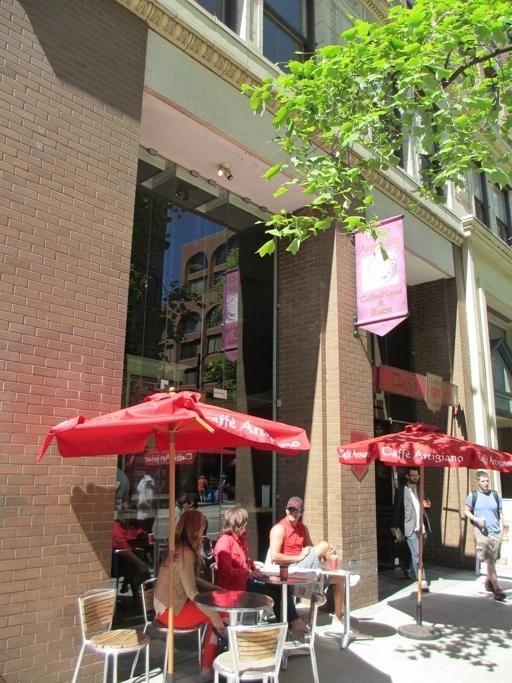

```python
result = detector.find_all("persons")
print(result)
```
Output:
[462,471,507,602]
[212,506,319,635]
[266,494,356,638]
[154,510,232,683]
[111,468,210,622]
[391,467,432,592]
[222,474,231,501]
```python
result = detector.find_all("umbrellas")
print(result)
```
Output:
[34,385,310,682]
[337,420,512,627]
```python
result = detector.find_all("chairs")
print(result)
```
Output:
[212,622,288,683]
[282,592,327,683]
[68,585,150,683]
[141,577,204,672]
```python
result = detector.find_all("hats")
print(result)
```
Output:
[284,495,304,512]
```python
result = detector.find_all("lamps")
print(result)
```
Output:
[216,164,233,180]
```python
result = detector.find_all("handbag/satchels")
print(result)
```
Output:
[315,571,336,612]
[212,621,230,656]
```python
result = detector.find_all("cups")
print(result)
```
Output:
[330,555,338,571]
[280,565,289,581]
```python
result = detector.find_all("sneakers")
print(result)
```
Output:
[330,619,358,633]
[484,577,494,592]
[494,588,506,600]
[421,580,429,590]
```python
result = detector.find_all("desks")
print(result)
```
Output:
[321,569,359,650]
[249,571,319,640]
[193,590,275,626]
